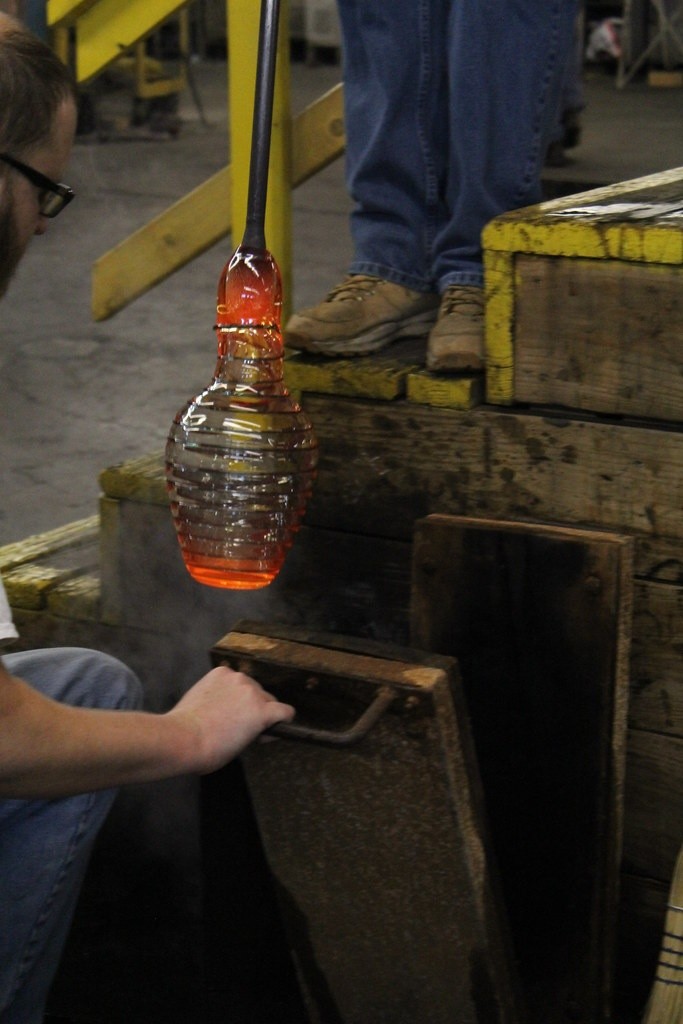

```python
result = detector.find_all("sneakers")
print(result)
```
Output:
[284,273,441,355]
[429,284,486,373]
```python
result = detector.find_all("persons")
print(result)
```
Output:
[0,8,298,1024]
[282,0,624,375]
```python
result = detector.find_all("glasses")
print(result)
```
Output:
[0,150,75,220]
